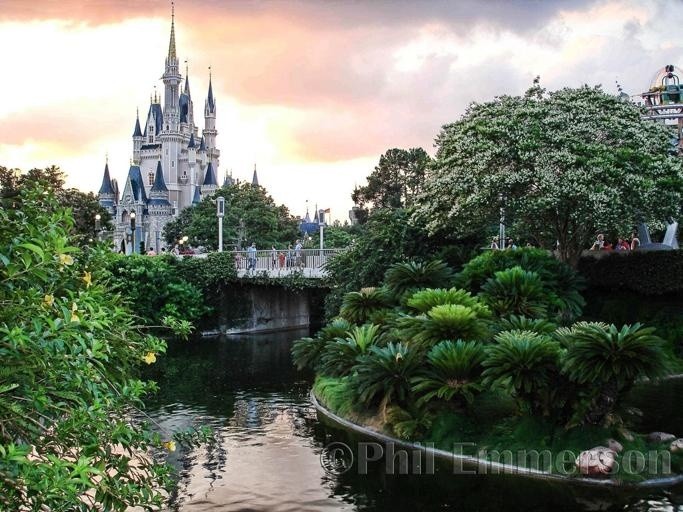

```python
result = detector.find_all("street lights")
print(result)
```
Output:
[130,212,136,253]
[317,208,325,265]
[94,214,101,242]
[215,195,224,253]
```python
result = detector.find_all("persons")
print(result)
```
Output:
[146,245,180,255]
[600,240,613,252]
[589,234,604,250]
[630,226,640,251]
[491,236,499,249]
[615,237,627,251]
[507,239,514,249]
[245,238,305,275]
